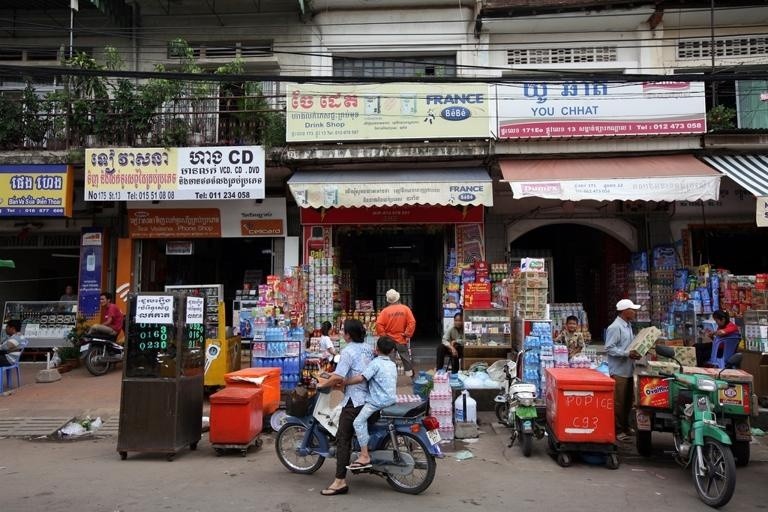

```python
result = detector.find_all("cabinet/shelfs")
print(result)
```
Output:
[503,266,548,353]
[462,307,512,398]
[653,248,675,328]
[628,251,650,336]
[1,301,76,359]
[376,278,413,313]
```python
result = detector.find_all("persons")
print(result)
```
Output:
[553,316,585,360]
[338,337,397,471]
[376,289,416,377]
[60,286,77,301]
[693,309,739,368]
[79,291,123,360]
[307,329,321,348]
[315,317,375,497]
[436,313,463,374]
[0,319,26,367]
[604,298,642,443]
[320,320,337,367]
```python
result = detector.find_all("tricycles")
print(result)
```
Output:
[631,344,759,507]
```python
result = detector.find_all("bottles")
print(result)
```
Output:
[432,372,449,392]
[438,425,454,440]
[488,274,508,282]
[431,409,452,425]
[429,387,452,408]
[491,263,508,274]
[254,327,304,342]
[554,344,570,368]
[303,358,330,384]
[540,335,554,398]
[253,317,267,329]
[532,323,550,336]
[280,375,302,392]
[524,336,540,396]
[251,354,306,374]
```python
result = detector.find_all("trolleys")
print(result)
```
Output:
[543,367,619,470]
[209,387,263,456]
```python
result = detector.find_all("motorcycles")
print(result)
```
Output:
[275,377,444,494]
[79,315,126,376]
[487,348,544,457]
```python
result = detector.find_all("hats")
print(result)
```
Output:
[615,297,643,313]
[384,288,402,304]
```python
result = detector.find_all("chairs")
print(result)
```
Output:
[0,339,29,395]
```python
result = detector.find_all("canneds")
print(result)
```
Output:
[337,312,380,324]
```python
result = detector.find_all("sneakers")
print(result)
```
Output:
[625,424,636,435]
[615,431,634,444]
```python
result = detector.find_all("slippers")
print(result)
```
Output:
[319,485,351,496]
[344,459,375,464]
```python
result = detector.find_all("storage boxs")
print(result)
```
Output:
[208,387,264,445]
[544,366,617,445]
[225,367,282,416]
